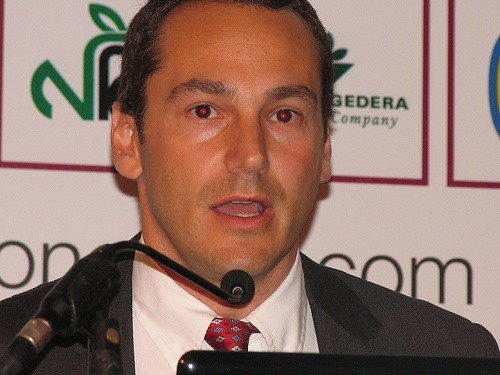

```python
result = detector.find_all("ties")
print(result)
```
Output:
[204,317,260,353]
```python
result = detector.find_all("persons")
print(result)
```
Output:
[1,1,500,375]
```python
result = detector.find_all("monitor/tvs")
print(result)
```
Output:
[176,350,500,374]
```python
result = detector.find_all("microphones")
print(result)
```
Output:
[112,239,255,306]
